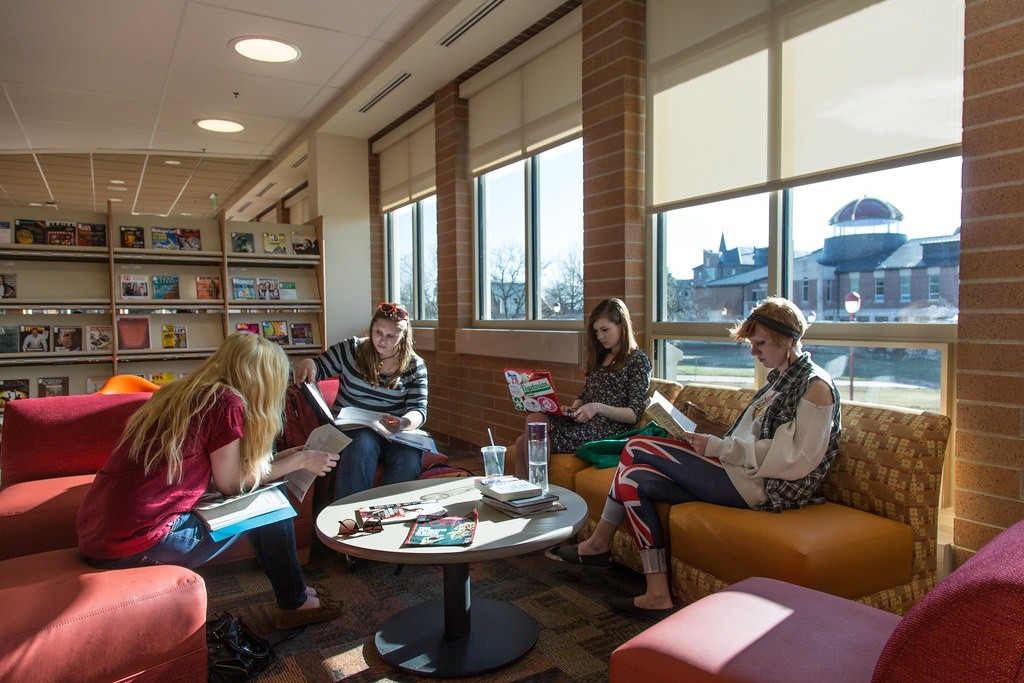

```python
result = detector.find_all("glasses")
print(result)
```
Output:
[379,301,409,318]
[337,516,384,534]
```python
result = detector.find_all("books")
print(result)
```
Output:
[643,390,697,441]
[474,475,567,518]
[303,382,438,454]
[404,509,478,546]
[0,219,320,418]
[359,500,447,523]
[193,480,298,542]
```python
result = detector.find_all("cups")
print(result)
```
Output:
[481,445,507,477]
[526,422,550,492]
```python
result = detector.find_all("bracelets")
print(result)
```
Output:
[573,408,577,410]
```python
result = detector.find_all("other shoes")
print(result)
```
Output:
[313,587,331,597]
[274,597,346,631]
[346,554,360,572]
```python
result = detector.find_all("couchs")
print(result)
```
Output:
[0,377,467,563]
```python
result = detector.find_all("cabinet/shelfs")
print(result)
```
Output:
[0,199,328,427]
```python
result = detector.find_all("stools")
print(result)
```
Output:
[0,550,207,683]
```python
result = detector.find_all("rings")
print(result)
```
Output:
[692,440,693,444]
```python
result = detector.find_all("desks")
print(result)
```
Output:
[316,477,589,676]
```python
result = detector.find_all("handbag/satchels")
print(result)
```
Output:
[575,420,668,469]
[206,610,276,683]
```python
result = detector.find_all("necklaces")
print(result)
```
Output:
[380,345,399,360]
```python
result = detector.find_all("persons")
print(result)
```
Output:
[0,275,14,296]
[22,328,47,352]
[557,298,841,621]
[303,239,311,249]
[262,282,274,292]
[515,298,652,479]
[208,281,218,297]
[76,331,341,629]
[296,302,428,499]
[58,331,81,351]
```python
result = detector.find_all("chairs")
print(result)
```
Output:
[504,378,684,490]
[610,518,1024,683]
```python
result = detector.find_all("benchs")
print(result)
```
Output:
[577,383,951,618]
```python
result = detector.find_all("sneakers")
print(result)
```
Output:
[609,596,673,623]
[544,543,613,569]
